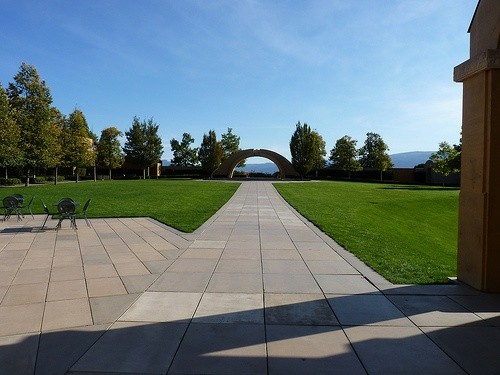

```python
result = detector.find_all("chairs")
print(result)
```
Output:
[0,192,93,233]
